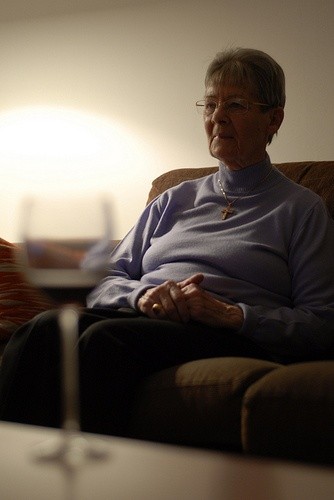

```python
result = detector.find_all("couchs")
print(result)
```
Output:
[147,160,334,458]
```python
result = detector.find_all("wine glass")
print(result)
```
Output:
[17,191,115,474]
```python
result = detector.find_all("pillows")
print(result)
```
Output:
[0,238,56,329]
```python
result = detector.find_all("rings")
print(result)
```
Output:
[152,304,161,313]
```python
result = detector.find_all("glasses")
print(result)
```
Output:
[195,98,270,116]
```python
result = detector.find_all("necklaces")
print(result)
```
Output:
[218,168,273,221]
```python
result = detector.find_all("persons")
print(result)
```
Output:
[0,48,334,439]
